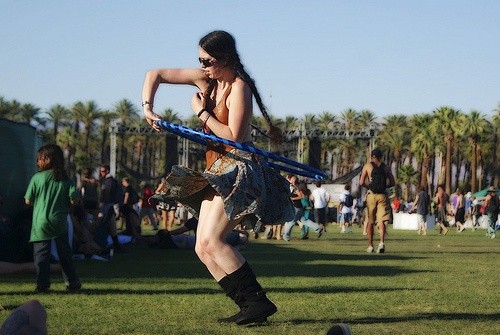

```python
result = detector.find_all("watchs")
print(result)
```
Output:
[142,100,151,106]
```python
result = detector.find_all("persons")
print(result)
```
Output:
[142,31,292,326]
[69,162,500,258]
[24,144,80,293]
[359,149,394,254]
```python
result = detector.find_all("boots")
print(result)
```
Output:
[217,276,249,324]
[226,262,277,324]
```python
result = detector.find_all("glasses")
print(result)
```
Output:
[198,58,218,67]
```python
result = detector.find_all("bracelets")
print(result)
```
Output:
[201,113,210,124]
[198,109,207,118]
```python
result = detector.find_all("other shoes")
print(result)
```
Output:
[67,283,81,294]
[316,227,323,238]
[276,235,281,240]
[377,243,385,253]
[366,247,374,252]
[254,231,258,239]
[459,228,465,232]
[34,286,47,293]
[272,233,275,238]
[443,229,448,235]
[301,232,308,240]
